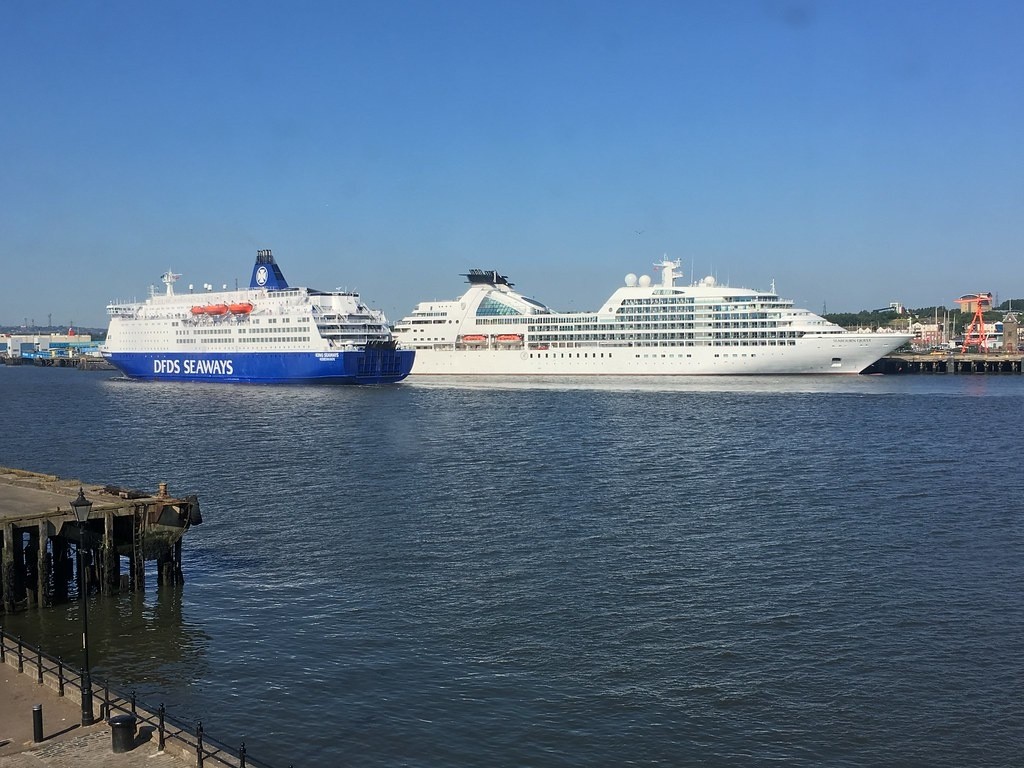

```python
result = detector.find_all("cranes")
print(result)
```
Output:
[952,292,992,354]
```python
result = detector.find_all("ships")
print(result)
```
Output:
[390,254,917,374]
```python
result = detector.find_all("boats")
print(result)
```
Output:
[100,249,416,385]
